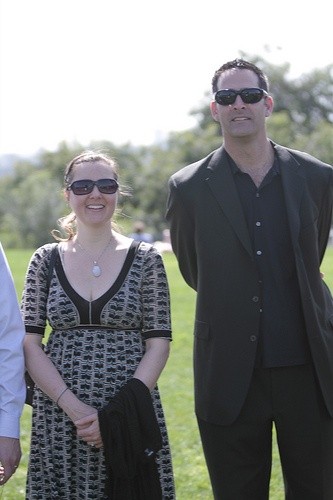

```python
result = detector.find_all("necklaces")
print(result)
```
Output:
[75,236,114,277]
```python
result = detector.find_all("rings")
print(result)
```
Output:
[14,465,18,468]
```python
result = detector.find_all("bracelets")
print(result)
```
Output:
[56,387,69,404]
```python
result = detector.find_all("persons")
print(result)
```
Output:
[0,241,26,485]
[166,58,333,500]
[22,150,175,500]
[128,223,153,244]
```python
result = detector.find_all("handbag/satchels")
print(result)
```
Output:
[24,243,59,406]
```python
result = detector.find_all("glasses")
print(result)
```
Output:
[213,87,269,106]
[66,178,119,195]
